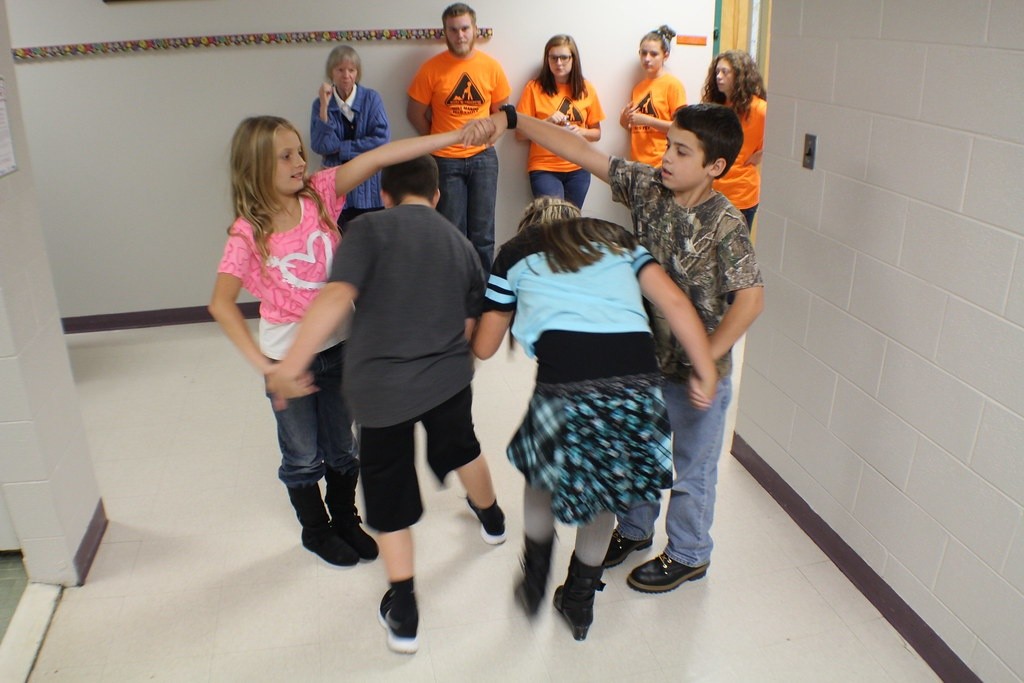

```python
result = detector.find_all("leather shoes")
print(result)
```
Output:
[626,553,710,593]
[602,530,654,570]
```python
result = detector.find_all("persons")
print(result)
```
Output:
[207,115,487,655]
[309,3,768,285]
[470,103,766,641]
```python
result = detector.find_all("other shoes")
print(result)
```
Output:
[465,494,506,545]
[380,588,420,654]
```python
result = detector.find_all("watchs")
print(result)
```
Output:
[498,104,517,129]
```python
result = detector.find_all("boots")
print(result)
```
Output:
[553,550,607,641]
[514,534,555,620]
[324,473,378,563]
[287,481,359,568]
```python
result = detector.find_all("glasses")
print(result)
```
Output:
[548,54,573,63]
